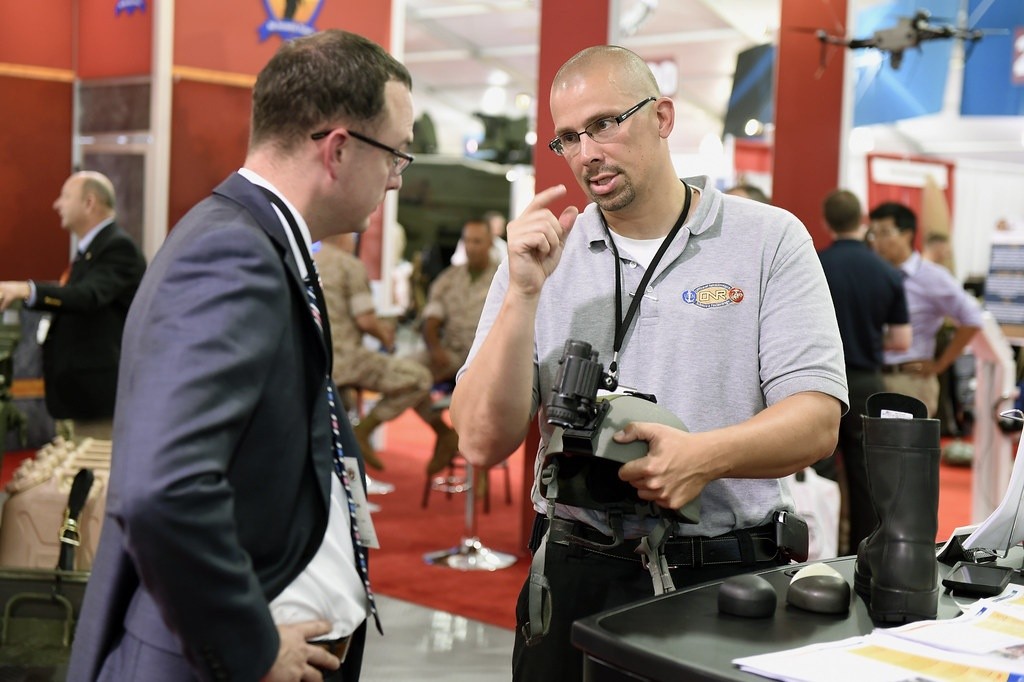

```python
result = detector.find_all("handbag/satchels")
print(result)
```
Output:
[0,592,77,682]
[936,533,1024,567]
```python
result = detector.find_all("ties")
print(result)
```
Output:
[69,250,82,271]
[304,277,384,635]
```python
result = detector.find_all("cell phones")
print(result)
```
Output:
[941,561,1014,595]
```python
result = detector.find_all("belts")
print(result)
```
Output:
[885,362,910,375]
[548,517,779,569]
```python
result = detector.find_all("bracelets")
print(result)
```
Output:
[24,280,31,303]
[937,362,944,374]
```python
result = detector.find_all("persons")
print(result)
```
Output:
[312,211,506,476]
[450,45,851,682]
[68,32,414,682]
[726,185,983,556]
[0,171,147,419]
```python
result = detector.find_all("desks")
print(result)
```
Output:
[567,541,1024,682]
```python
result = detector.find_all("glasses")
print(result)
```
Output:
[311,129,414,176]
[548,97,657,158]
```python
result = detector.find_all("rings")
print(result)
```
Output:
[0,293,2,300]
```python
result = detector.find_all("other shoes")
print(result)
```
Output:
[361,450,383,470]
[427,430,459,475]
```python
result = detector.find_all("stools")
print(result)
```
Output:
[334,384,517,572]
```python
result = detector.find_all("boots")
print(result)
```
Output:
[853,392,940,623]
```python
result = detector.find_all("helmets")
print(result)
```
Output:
[538,396,703,526]
